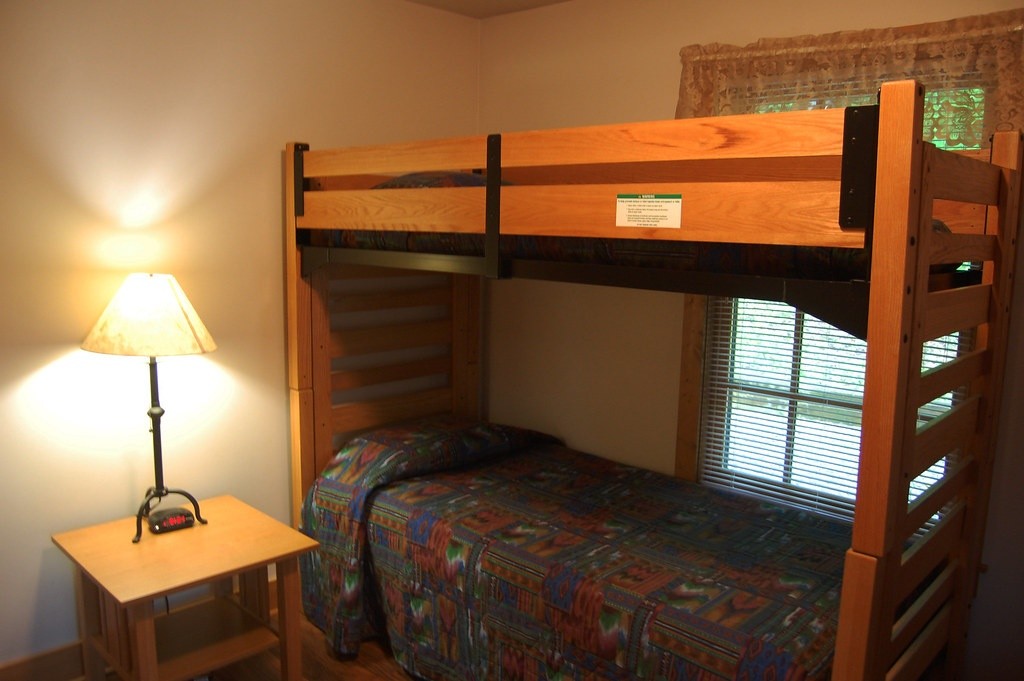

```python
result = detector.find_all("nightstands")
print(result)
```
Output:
[52,494,319,681]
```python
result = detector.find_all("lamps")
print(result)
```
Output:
[82,270,220,546]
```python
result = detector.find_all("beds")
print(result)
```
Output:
[281,78,1024,681]
